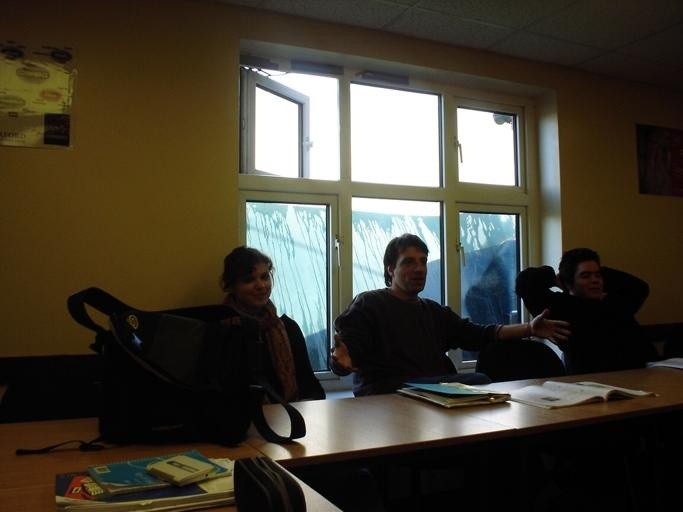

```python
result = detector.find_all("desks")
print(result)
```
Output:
[0,365,682,511]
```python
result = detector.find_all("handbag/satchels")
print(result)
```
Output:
[68,287,268,446]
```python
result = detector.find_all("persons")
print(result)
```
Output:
[221,247,326,400]
[330,234,572,397]
[516,248,661,372]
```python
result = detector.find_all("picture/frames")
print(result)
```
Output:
[635,122,683,198]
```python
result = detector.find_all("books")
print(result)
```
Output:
[511,381,661,410]
[54,449,239,512]
[396,381,512,410]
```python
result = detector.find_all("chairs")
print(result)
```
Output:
[476,335,573,382]
